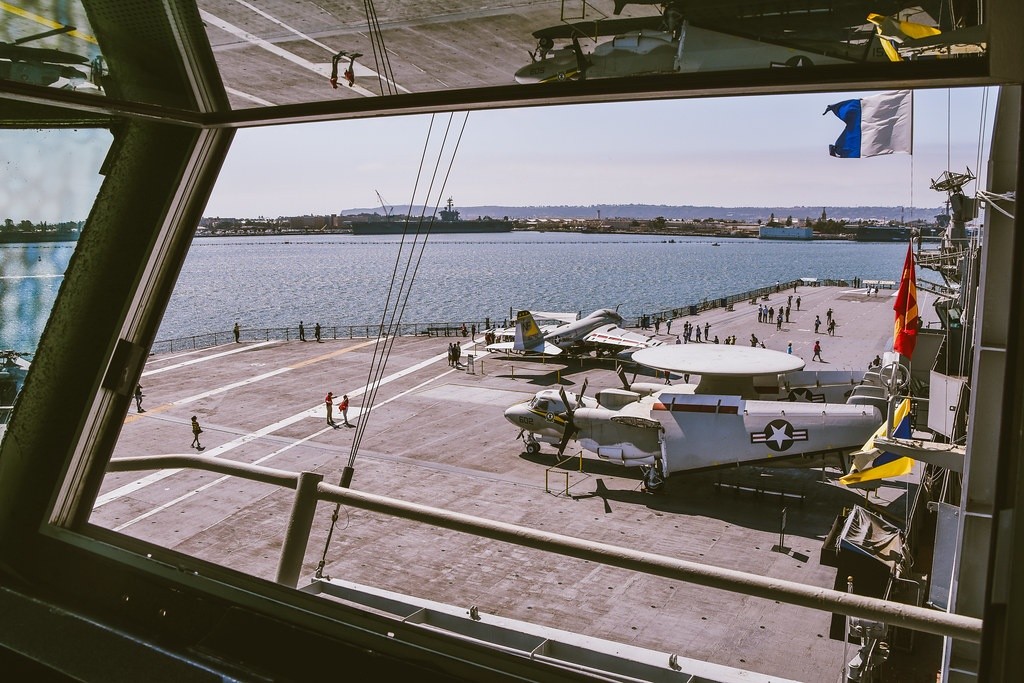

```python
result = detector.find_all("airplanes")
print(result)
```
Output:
[503,382,666,466]
[480,305,669,356]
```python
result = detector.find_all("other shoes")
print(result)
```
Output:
[191,444,195,447]
[327,421,335,424]
[343,421,348,424]
[197,443,201,445]
[137,406,141,412]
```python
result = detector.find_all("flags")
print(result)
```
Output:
[839,394,915,485]
[867,5,942,62]
[892,239,919,362]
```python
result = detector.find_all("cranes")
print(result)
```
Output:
[375,190,395,218]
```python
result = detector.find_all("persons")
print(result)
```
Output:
[461,323,469,337]
[299,321,307,342]
[641,279,880,385]
[325,392,337,425]
[234,323,241,343]
[315,323,321,342]
[135,382,147,413]
[330,51,363,89]
[190,415,203,448]
[341,394,349,425]
[472,325,476,343]
[447,342,462,367]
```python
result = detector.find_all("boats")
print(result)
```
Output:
[438,196,460,221]
[919,201,953,243]
[758,225,817,242]
[857,223,911,241]
